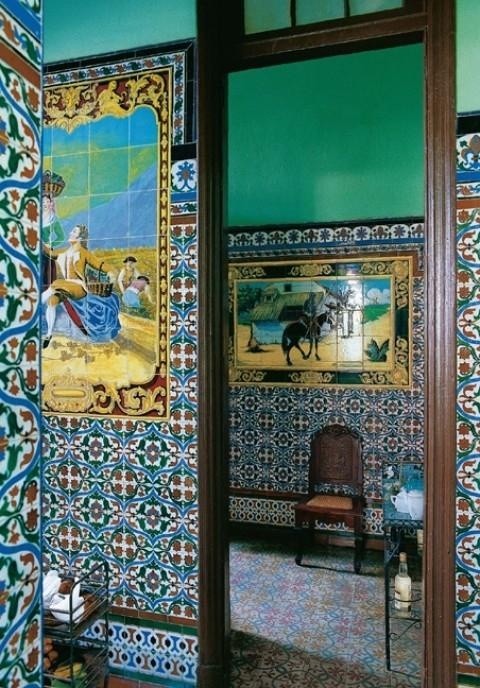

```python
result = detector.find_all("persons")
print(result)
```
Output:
[116,253,141,295]
[42,191,65,290]
[299,289,319,324]
[121,272,158,310]
[38,222,119,352]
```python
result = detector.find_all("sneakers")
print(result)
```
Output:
[42,334,53,350]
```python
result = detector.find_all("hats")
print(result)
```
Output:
[122,256,138,263]
[136,276,151,285]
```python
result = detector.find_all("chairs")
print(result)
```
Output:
[293,424,365,573]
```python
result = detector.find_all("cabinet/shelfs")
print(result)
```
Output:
[43,563,111,688]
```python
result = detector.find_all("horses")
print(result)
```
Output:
[282,310,333,366]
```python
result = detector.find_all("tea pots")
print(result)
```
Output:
[389,486,407,514]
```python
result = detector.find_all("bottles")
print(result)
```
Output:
[404,488,424,520]
[392,550,414,619]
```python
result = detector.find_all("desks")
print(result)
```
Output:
[381,462,426,671]
